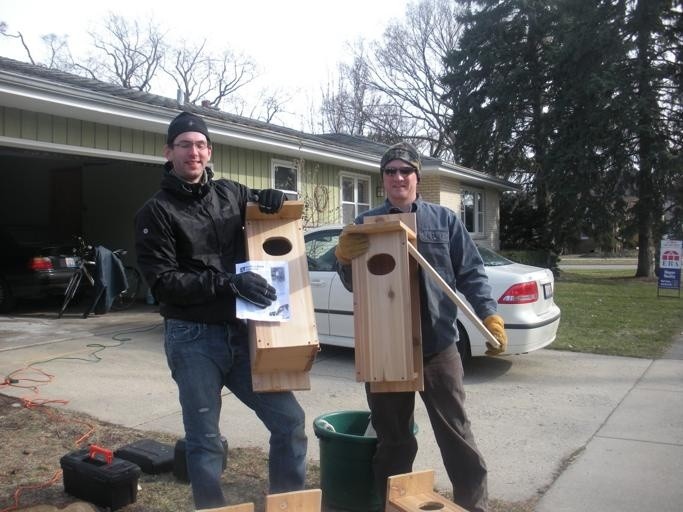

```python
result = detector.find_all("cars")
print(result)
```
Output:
[0,241,82,313]
[304,224,561,366]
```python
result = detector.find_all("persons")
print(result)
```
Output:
[335,141,508,511]
[134,112,307,509]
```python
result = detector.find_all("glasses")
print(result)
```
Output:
[385,166,416,176]
[173,142,209,150]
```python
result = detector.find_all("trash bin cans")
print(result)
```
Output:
[313,410,419,512]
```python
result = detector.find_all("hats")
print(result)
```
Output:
[380,141,421,182]
[168,112,210,144]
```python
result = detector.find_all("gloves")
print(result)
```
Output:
[335,225,370,262]
[254,189,285,215]
[483,315,508,356]
[228,271,277,309]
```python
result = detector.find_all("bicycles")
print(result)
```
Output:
[58,231,142,319]
